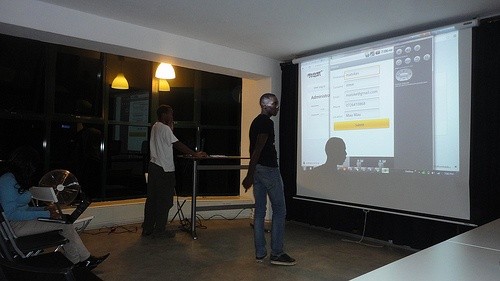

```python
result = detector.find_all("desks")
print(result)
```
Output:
[446,219,500,252]
[347,241,500,281]
[177,153,272,241]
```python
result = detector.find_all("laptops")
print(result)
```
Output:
[40,198,94,224]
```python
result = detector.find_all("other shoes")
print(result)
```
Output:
[84,252,110,272]
[141,231,176,239]
[256,253,268,263]
[270,253,297,265]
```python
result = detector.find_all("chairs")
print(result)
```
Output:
[0,204,71,281]
[30,186,95,239]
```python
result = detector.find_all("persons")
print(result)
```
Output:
[77,123,102,199]
[141,105,205,238]
[242,93,296,266]
[0,160,111,272]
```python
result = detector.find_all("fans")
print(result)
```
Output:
[38,168,81,210]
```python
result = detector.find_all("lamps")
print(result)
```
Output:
[154,62,177,81]
[110,72,129,90]
[158,79,171,92]
